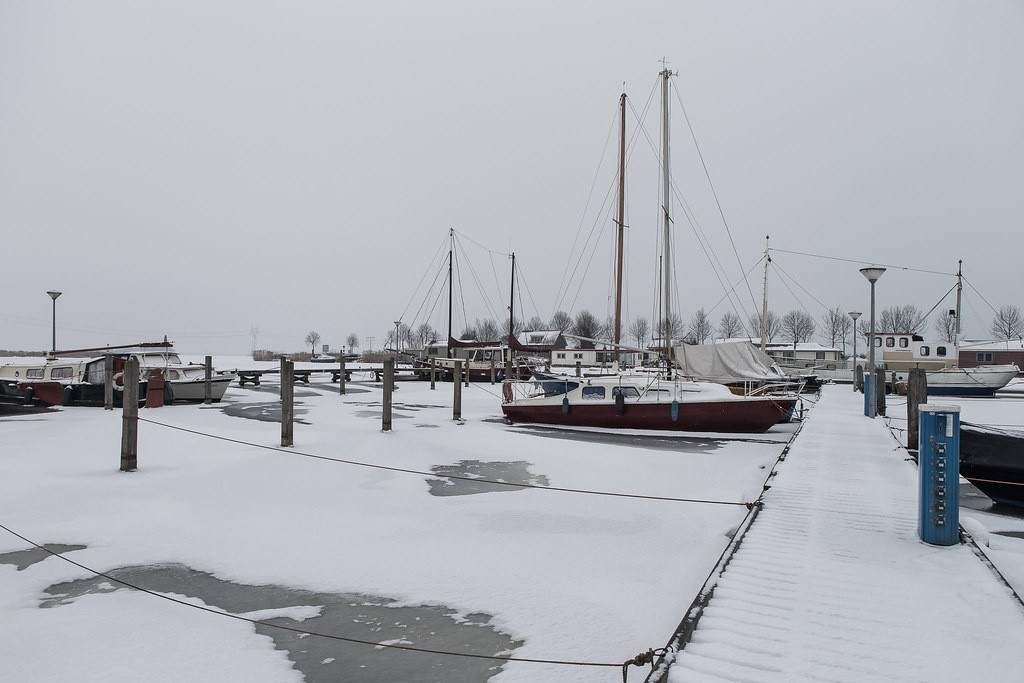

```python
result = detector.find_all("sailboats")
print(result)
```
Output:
[529,92,830,399]
[863,260,1020,398]
[493,66,802,434]
[381,227,536,382]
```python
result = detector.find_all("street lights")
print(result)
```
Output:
[394,321,402,369]
[859,264,887,418]
[46,289,62,352]
[847,311,863,392]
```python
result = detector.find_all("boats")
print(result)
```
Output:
[0,336,236,408]
[311,354,336,363]
[958,420,1024,509]
